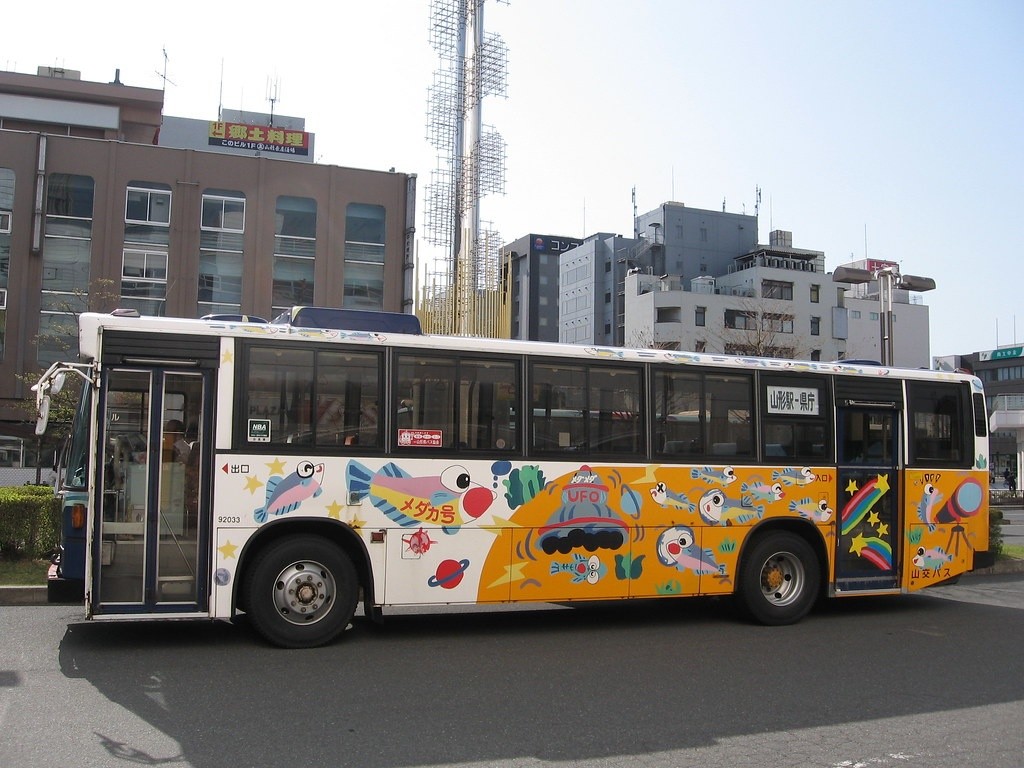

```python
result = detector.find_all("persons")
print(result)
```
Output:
[990,469,995,484]
[398,379,414,409]
[1007,471,1016,490]
[1004,468,1010,486]
[162,420,190,464]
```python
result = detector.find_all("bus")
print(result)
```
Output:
[30,304,996,649]
[395,396,895,455]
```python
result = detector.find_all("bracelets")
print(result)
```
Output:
[400,399,404,406]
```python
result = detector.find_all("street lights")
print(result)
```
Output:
[832,265,937,367]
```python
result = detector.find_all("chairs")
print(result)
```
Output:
[663,441,788,457]
[450,442,466,448]
[287,432,357,445]
